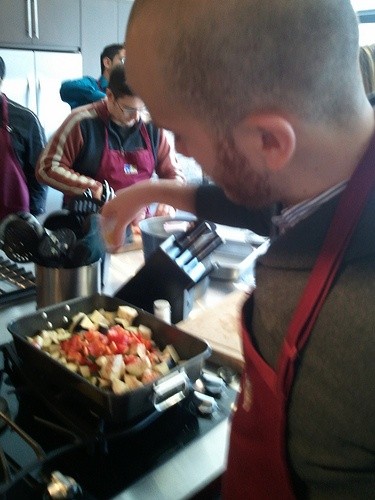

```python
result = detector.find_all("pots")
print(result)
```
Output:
[7,292,213,428]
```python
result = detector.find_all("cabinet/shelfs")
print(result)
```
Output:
[0,0,134,81]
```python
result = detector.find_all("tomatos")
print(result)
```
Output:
[60,324,158,372]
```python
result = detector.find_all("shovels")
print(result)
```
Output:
[70,195,105,240]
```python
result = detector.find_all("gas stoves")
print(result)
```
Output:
[0,254,242,500]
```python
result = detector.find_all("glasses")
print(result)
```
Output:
[117,55,126,64]
[116,98,147,119]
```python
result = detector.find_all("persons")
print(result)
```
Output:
[101,0,375,499]
[59,44,125,109]
[0,58,50,218]
[36,65,186,230]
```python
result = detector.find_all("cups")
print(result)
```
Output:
[34,255,102,311]
[138,216,209,299]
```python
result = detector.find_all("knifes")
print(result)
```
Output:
[169,221,225,271]
